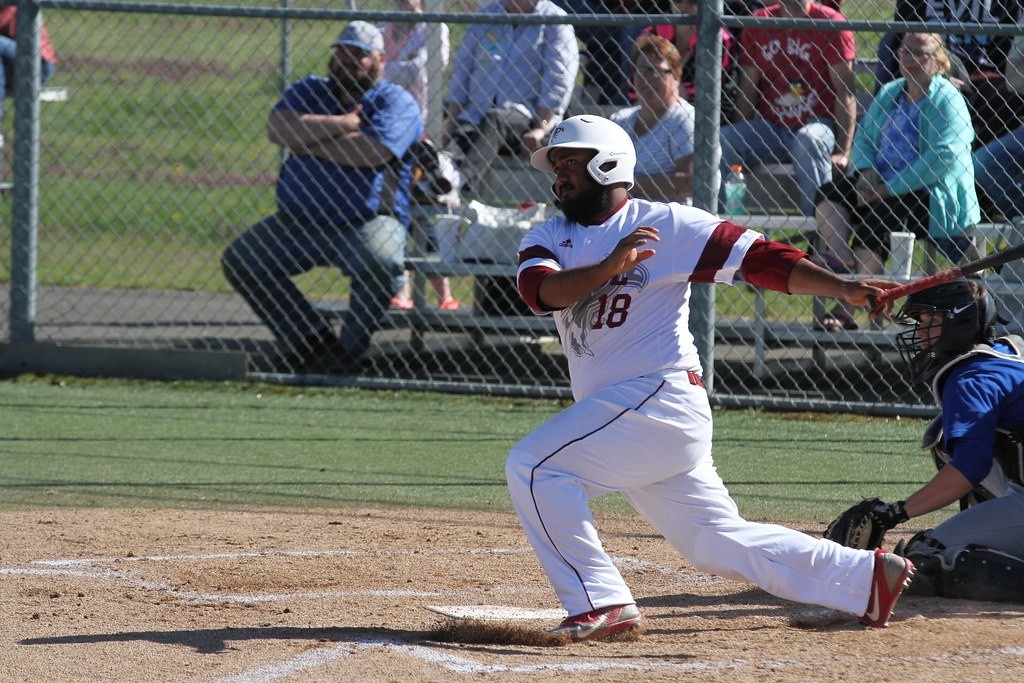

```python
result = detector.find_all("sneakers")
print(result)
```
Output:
[548,597,641,641]
[859,547,916,628]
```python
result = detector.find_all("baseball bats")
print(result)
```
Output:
[866,244,1024,312]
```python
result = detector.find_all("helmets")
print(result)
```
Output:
[531,115,637,202]
[895,277,996,384]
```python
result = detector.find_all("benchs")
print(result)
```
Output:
[291,61,1022,397]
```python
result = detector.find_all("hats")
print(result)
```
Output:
[330,19,385,55]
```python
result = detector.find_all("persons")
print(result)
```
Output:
[375,0,460,311]
[505,115,915,644]
[555,0,1024,329]
[827,278,1024,605]
[221,20,422,377]
[0,6,56,102]
[405,0,580,205]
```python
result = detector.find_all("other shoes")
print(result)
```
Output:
[320,341,361,374]
[438,299,462,310]
[273,329,336,372]
[389,295,414,309]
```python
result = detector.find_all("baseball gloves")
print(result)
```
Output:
[822,497,899,551]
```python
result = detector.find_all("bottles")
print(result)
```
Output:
[725,165,746,215]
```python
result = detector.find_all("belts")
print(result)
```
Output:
[688,371,704,387]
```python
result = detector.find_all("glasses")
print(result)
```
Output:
[898,48,936,60]
[633,65,671,78]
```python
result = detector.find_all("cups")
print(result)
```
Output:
[891,233,916,277]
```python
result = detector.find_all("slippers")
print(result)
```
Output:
[813,313,859,332]
[807,252,854,272]
